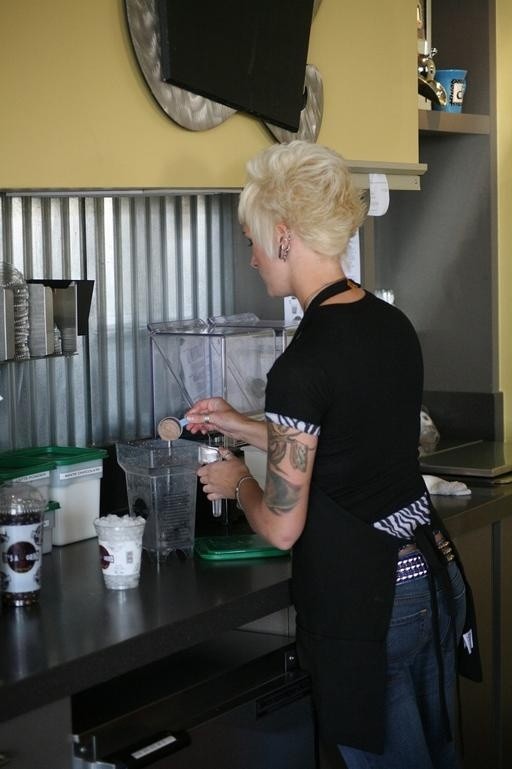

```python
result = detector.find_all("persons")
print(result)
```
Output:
[182,137,483,769]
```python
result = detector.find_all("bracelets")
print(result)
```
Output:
[234,475,253,508]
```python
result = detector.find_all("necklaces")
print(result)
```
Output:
[304,280,334,310]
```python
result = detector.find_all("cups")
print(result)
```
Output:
[433,69,468,113]
[93,518,146,590]
[0,483,46,608]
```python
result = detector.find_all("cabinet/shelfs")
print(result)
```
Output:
[450,512,511,769]
[1,2,500,194]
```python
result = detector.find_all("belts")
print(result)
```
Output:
[395,541,456,587]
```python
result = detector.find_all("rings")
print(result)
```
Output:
[223,451,232,458]
[204,414,210,423]
